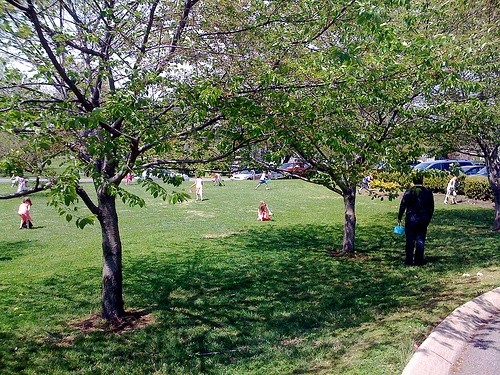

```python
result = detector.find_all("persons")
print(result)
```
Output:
[444,175,460,205]
[358,174,373,194]
[19,197,32,229]
[258,201,272,221]
[211,172,222,185]
[126,172,133,184]
[257,171,270,191]
[190,173,204,200]
[398,172,435,267]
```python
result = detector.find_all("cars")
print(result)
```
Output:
[233,155,315,181]
[408,157,489,176]
